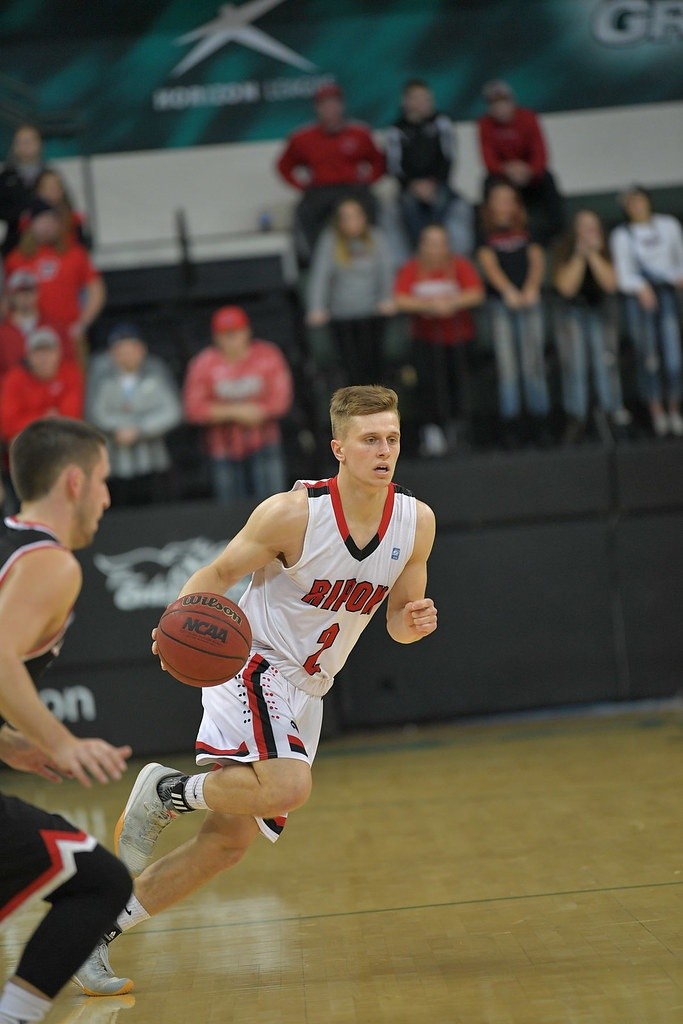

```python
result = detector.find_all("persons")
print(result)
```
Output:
[73,385,437,995]
[0,418,135,1024]
[0,81,683,504]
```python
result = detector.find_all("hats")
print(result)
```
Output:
[9,270,35,289]
[317,84,340,99]
[485,85,509,101]
[216,309,246,331]
[27,329,59,352]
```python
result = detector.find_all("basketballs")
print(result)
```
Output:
[156,592,254,688]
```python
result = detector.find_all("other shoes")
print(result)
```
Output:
[425,410,683,454]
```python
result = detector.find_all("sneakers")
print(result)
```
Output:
[59,996,136,1024]
[72,943,134,996]
[114,763,182,878]
